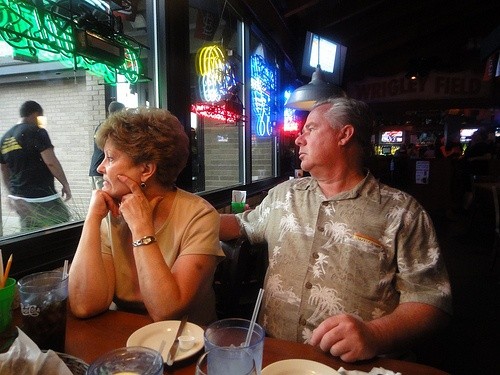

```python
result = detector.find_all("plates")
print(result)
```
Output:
[261,359,342,375]
[126,320,205,363]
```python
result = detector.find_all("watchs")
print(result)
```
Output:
[131,235,157,246]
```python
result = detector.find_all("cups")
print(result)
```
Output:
[195,347,257,375]
[0,277,16,333]
[203,318,265,375]
[17,271,69,354]
[231,202,245,214]
[86,346,163,375]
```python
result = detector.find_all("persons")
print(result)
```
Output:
[437,128,497,176]
[88,101,125,190]
[0,100,72,233]
[68,106,226,325]
[219,97,451,362]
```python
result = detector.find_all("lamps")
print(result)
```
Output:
[284,36,337,111]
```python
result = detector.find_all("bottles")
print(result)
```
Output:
[374,144,399,155]
[462,143,467,151]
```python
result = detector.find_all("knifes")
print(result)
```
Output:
[166,314,189,366]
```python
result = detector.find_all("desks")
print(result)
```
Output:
[8,304,453,375]
